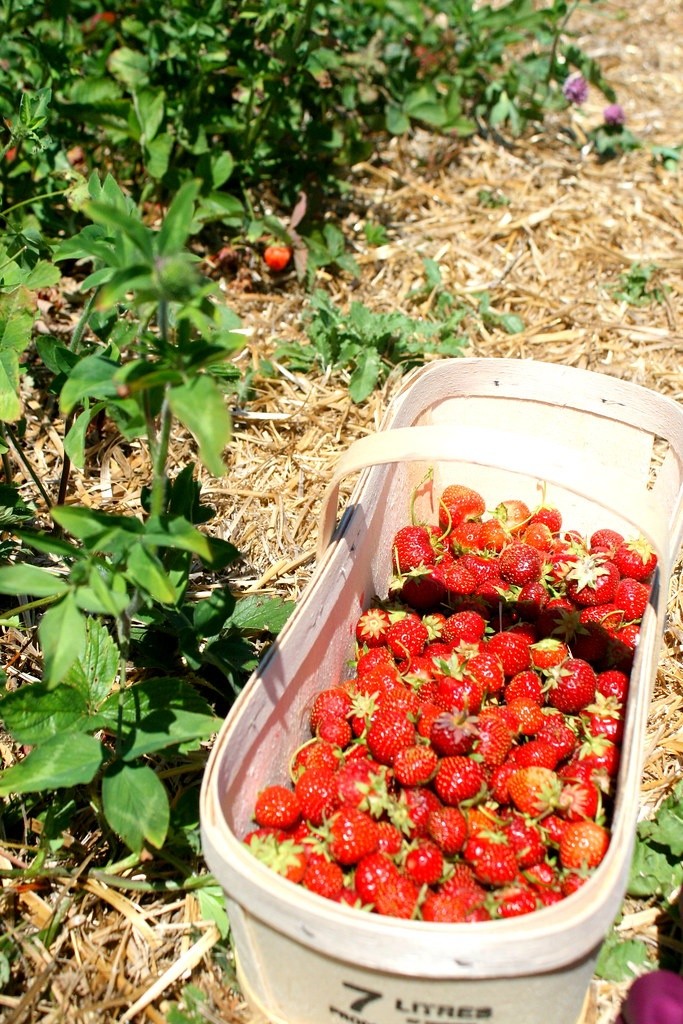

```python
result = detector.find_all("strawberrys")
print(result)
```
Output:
[264,241,291,272]
[244,487,658,927]
